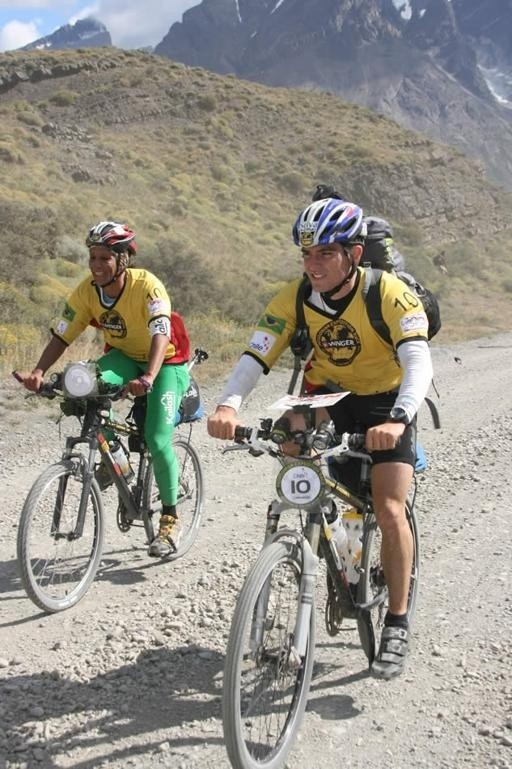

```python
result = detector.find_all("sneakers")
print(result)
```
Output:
[94,448,134,490]
[300,520,326,558]
[148,515,185,558]
[370,625,411,681]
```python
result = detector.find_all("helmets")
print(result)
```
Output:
[290,196,368,252]
[85,220,138,256]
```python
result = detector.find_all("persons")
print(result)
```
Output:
[206,195,435,679]
[20,220,192,559]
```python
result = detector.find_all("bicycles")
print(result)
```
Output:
[13,361,205,613]
[207,393,422,769]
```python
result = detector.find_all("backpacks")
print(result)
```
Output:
[357,215,442,343]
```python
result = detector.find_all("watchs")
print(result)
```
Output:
[389,406,410,428]
